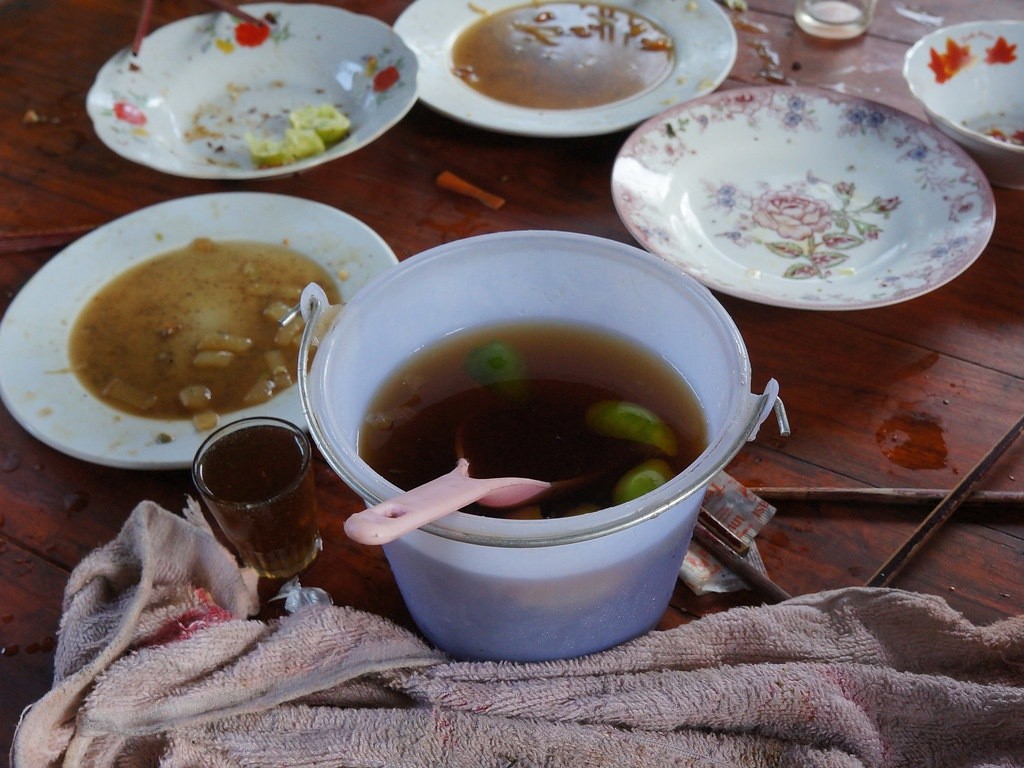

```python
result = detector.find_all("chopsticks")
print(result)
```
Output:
[695,412,1024,603]
[132,0,267,55]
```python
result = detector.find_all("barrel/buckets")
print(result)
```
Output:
[280,229,791,663]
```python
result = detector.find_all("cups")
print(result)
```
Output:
[191,417,322,580]
[793,0,878,39]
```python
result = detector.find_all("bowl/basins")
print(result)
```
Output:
[900,18,1023,188]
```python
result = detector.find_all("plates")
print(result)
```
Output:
[86,3,424,181]
[392,0,739,138]
[0,190,402,471]
[608,86,998,313]
